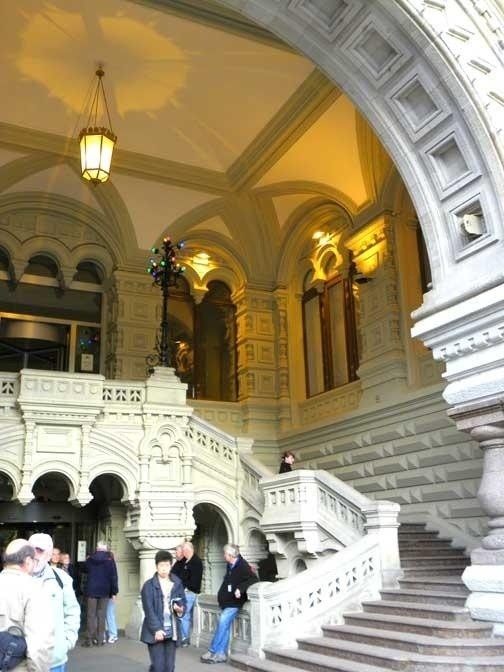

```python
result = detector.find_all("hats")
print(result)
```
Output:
[29,533,53,552]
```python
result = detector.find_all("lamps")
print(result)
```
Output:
[78,66,117,187]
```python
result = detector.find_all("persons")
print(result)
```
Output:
[138,550,188,672]
[0,538,56,672]
[277,450,296,473]
[47,547,61,567]
[199,542,259,664]
[58,551,79,601]
[176,542,203,648]
[102,543,121,645]
[81,539,119,649]
[26,532,82,672]
[171,545,188,648]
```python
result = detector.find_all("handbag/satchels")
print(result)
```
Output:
[0,631,27,672]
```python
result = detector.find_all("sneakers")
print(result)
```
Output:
[82,641,92,647]
[200,652,227,663]
[108,635,118,643]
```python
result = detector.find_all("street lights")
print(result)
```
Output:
[143,236,187,376]
